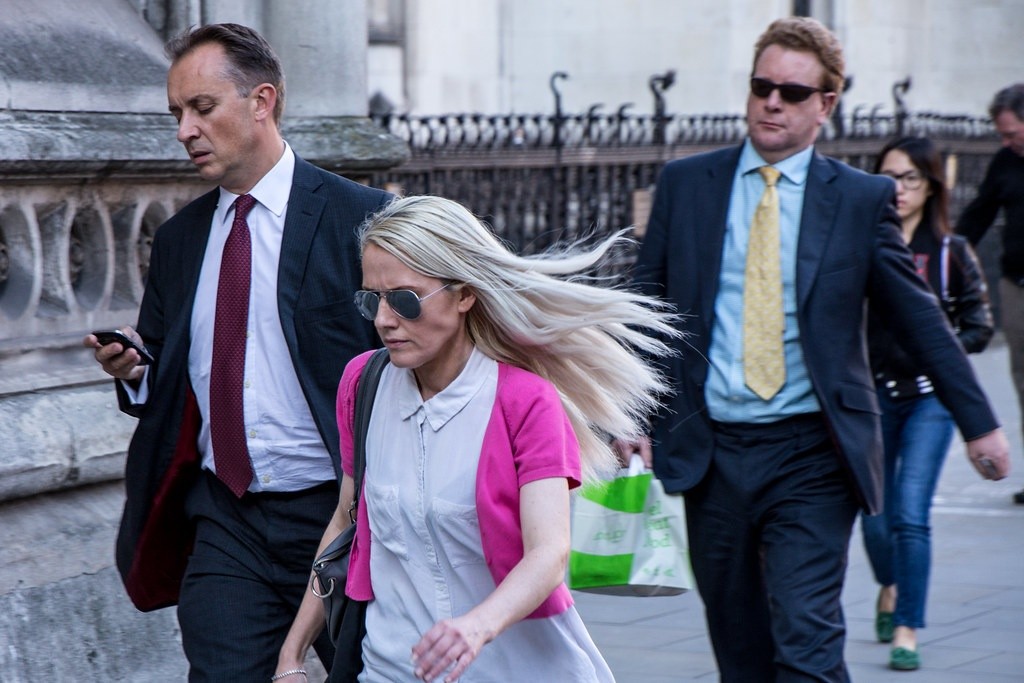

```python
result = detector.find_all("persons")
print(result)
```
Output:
[275,197,699,683]
[951,84,1024,506]
[861,136,995,671]
[609,16,1010,683]
[84,22,404,683]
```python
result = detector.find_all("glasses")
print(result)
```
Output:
[355,282,454,319]
[750,77,826,105]
[882,168,929,188]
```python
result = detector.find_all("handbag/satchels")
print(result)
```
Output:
[321,347,393,683]
[565,455,691,599]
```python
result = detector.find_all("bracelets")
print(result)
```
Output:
[271,670,307,681]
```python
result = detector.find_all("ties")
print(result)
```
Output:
[209,193,262,495]
[743,165,786,401]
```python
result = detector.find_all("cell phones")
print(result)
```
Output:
[91,329,154,365]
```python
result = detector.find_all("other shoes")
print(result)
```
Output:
[876,585,898,642]
[890,642,919,668]
